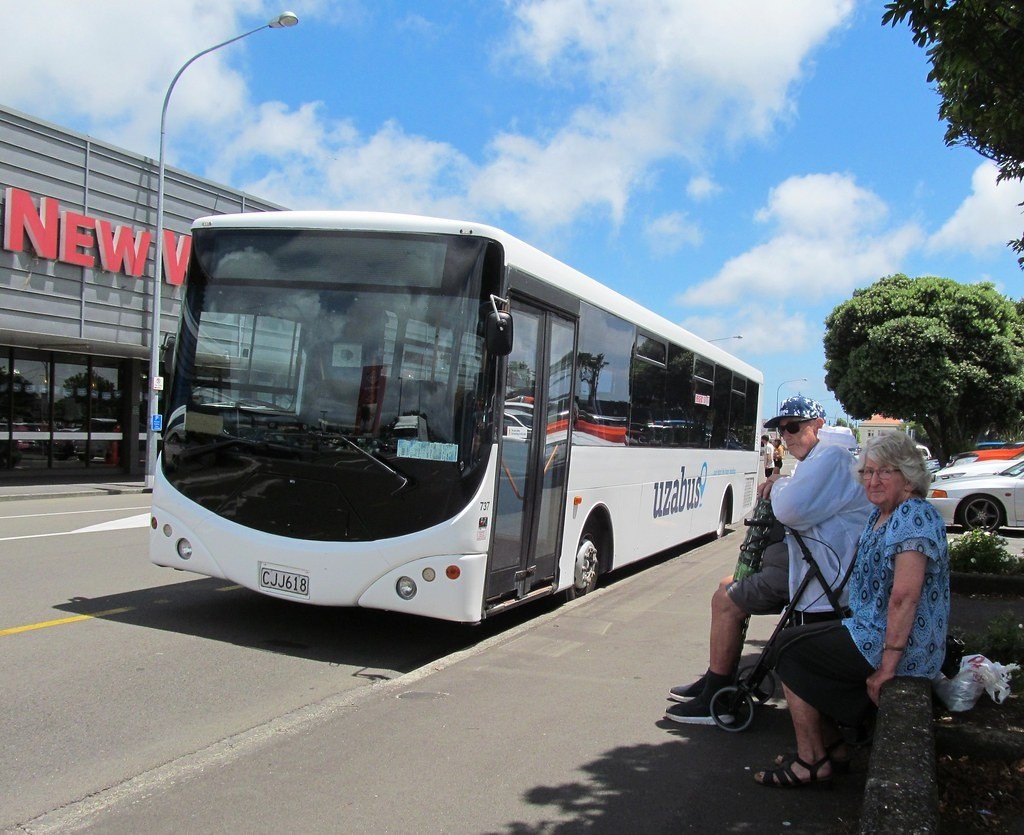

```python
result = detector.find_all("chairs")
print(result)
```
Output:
[302,379,367,433]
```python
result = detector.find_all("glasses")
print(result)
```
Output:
[778,418,817,437]
[859,467,901,480]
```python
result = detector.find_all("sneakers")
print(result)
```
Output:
[664,669,738,726]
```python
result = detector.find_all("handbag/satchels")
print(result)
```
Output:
[932,634,1011,712]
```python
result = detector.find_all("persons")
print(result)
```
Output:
[772,437,785,475]
[759,434,776,478]
[665,394,875,726]
[753,429,950,785]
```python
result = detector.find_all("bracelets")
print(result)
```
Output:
[882,641,906,653]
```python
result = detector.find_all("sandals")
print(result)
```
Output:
[753,751,834,788]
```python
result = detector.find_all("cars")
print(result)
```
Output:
[51,415,118,461]
[916,439,1024,530]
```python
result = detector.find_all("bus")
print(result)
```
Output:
[143,208,763,628]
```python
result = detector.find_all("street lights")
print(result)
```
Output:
[709,335,744,343]
[775,378,808,439]
[143,11,302,482]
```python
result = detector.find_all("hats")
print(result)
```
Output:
[764,394,829,428]
